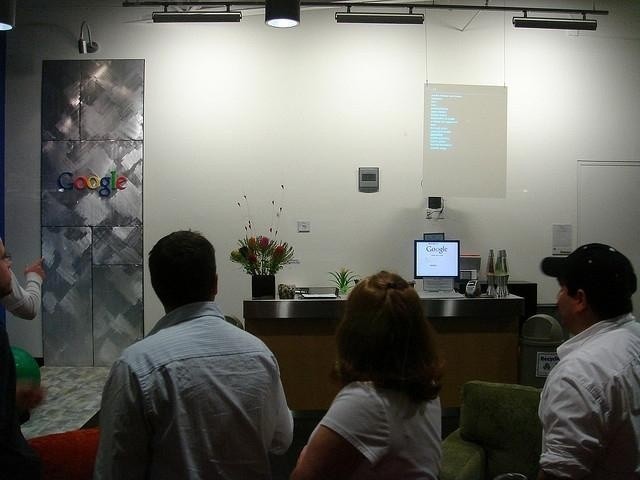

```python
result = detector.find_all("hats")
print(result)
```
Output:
[542,242,637,295]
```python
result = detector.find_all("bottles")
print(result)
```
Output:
[487,248,508,295]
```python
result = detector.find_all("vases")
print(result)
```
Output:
[252,275,275,299]
[340,288,346,295]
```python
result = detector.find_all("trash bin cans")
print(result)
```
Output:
[520,314,565,388]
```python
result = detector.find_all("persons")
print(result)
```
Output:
[93,229,295,478]
[1,239,45,480]
[1,253,46,320]
[534,242,640,479]
[288,271,448,479]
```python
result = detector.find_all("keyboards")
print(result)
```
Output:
[418,291,464,298]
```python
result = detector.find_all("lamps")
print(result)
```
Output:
[122,1,609,31]
[0,0,16,31]
[78,21,99,54]
[265,0,300,28]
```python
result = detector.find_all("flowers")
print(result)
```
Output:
[229,234,300,276]
[328,267,356,287]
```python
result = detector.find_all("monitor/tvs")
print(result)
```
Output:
[413,239,461,279]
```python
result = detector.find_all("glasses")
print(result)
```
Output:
[0,252,12,262]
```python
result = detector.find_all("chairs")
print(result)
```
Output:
[439,379,542,480]
[26,426,100,480]
